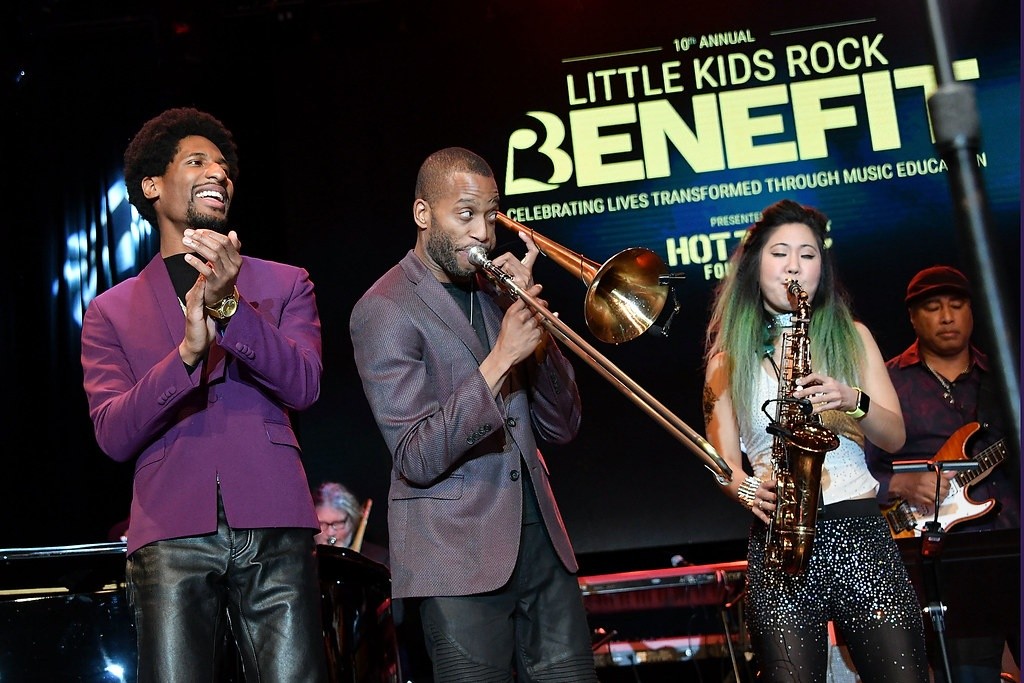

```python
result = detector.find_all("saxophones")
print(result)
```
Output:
[761,279,841,574]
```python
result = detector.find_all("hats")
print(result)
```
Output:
[905,264,980,308]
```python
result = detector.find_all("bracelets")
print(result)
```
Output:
[738,475,763,509]
[846,386,870,420]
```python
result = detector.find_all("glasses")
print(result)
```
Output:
[320,513,349,530]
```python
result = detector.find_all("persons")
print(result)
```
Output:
[313,482,365,549]
[702,198,931,683]
[866,264,1023,682]
[351,144,599,683]
[80,108,336,683]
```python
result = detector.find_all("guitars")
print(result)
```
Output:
[880,422,1012,544]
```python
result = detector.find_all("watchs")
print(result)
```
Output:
[204,285,239,318]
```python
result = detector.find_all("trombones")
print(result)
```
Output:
[468,210,732,487]
